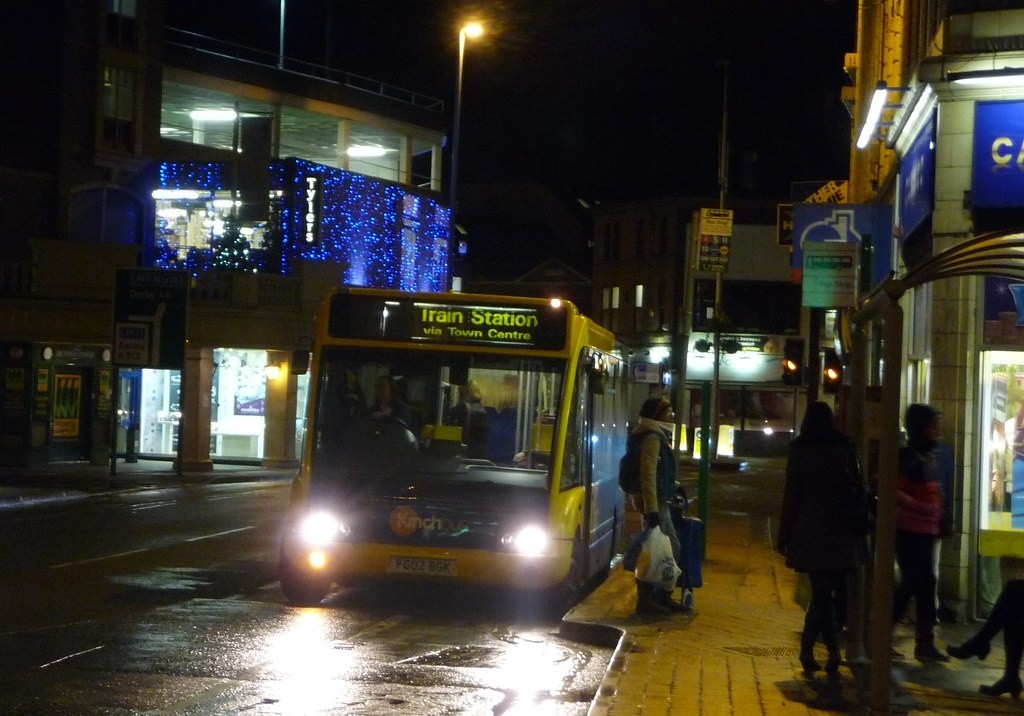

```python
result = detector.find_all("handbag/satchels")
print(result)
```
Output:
[635,529,682,591]
[623,523,679,571]
[618,431,663,495]
[846,436,879,535]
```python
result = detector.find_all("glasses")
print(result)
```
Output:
[662,411,675,418]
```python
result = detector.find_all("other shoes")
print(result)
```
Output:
[914,641,949,661]
[890,648,904,658]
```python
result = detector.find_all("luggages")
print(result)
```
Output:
[663,485,704,610]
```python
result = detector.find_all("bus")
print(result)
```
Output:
[280,287,630,611]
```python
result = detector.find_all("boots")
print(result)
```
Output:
[656,587,692,613]
[823,599,842,673]
[636,580,671,616]
[799,603,822,672]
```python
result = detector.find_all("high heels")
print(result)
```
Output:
[981,672,1023,699]
[947,638,990,660]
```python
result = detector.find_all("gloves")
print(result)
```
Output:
[645,512,660,527]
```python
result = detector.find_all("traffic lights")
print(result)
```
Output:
[783,339,802,387]
[824,348,843,395]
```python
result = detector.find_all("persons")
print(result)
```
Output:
[364,376,410,427]
[947,577,1024,698]
[889,404,949,662]
[460,385,488,447]
[777,400,874,671]
[628,397,688,613]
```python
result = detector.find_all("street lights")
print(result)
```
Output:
[453,21,485,294]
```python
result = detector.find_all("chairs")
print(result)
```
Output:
[527,416,556,454]
